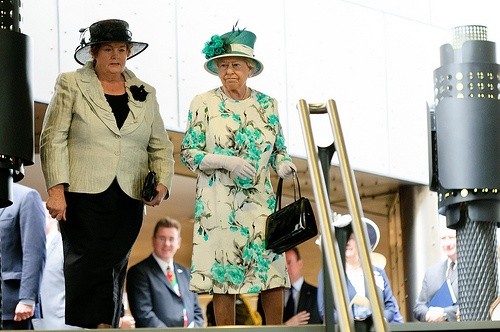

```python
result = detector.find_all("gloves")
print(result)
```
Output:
[200,153,256,180]
[275,161,298,179]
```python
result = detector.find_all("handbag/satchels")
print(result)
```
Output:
[265,165,317,254]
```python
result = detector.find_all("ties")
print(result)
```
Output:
[167,265,187,326]
[281,285,295,320]
[448,262,455,283]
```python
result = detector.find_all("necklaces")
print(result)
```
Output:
[240,85,249,101]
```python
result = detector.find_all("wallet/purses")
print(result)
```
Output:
[141,170,158,201]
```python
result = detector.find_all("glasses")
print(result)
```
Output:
[156,235,178,241]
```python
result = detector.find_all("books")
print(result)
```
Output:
[425,279,457,309]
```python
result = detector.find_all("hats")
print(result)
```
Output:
[315,211,380,256]
[200,19,264,73]
[74,19,148,64]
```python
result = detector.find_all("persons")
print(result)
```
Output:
[0,182,47,330]
[39,18,175,328]
[127,217,205,328]
[257,249,323,325]
[31,200,136,329]
[316,217,402,323]
[412,223,457,322]
[180,29,297,326]
